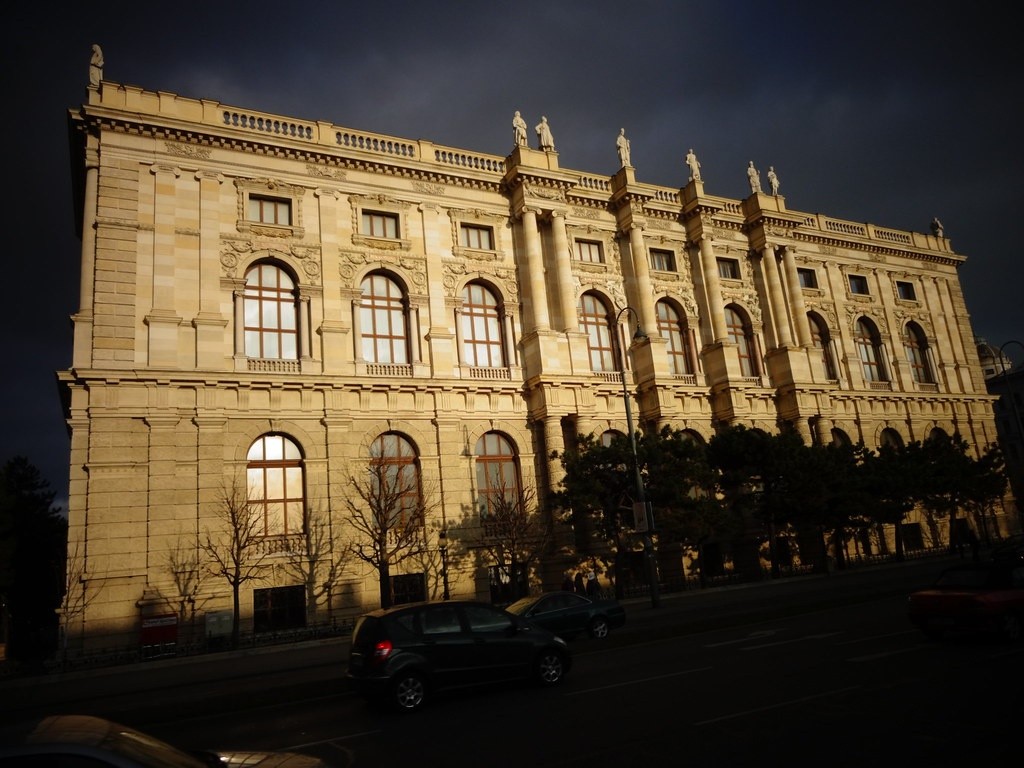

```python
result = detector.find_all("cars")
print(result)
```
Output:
[910,560,1023,623]
[508,593,615,641]
[2,715,322,768]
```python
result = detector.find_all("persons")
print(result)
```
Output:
[747,161,762,191]
[767,166,779,194]
[513,111,528,146]
[561,572,602,596]
[686,149,701,177]
[90,45,103,85]
[616,128,631,167]
[535,116,555,147]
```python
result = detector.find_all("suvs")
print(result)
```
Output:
[349,597,566,712]
